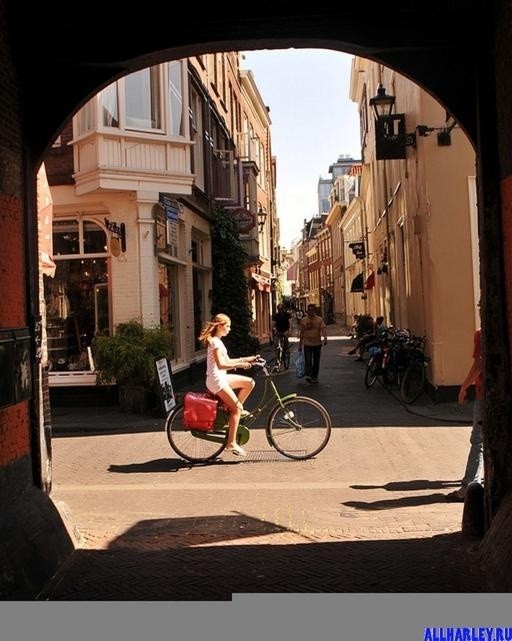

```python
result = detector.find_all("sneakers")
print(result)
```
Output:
[305,377,319,384]
[240,409,254,417]
[224,443,246,457]
[445,489,465,501]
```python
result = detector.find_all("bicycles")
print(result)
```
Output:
[165,353,332,463]
[365,326,431,404]
[272,328,291,370]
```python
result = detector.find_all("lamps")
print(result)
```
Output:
[368,84,417,162]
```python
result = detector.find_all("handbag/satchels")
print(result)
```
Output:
[182,391,218,431]
[295,351,305,378]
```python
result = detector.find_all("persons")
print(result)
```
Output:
[298,304,328,384]
[269,303,291,369]
[444,299,485,504]
[197,314,260,458]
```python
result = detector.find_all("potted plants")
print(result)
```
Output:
[84,321,175,414]
